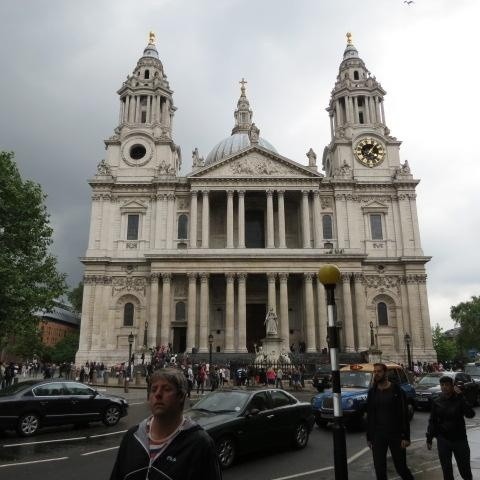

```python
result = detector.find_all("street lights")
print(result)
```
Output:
[326,335,331,363]
[144,320,148,352]
[317,264,349,480]
[404,332,412,371]
[208,331,213,386]
[128,331,134,383]
[369,321,375,347]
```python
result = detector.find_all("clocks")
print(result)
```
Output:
[355,137,387,169]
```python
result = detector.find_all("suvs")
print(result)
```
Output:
[460,362,480,389]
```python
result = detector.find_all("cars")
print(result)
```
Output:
[415,370,480,409]
[0,378,129,437]
[312,364,348,393]
[311,362,416,433]
[183,386,316,470]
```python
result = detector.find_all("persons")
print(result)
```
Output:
[366,363,415,480]
[248,122,260,140]
[109,369,224,480]
[159,160,167,174]
[99,159,107,174]
[412,359,465,372]
[306,147,317,165]
[344,159,352,174]
[401,160,409,169]
[2,361,135,392]
[426,376,475,480]
[193,147,199,164]
[265,307,279,333]
[142,346,306,392]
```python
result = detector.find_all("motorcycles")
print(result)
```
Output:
[115,358,128,378]
